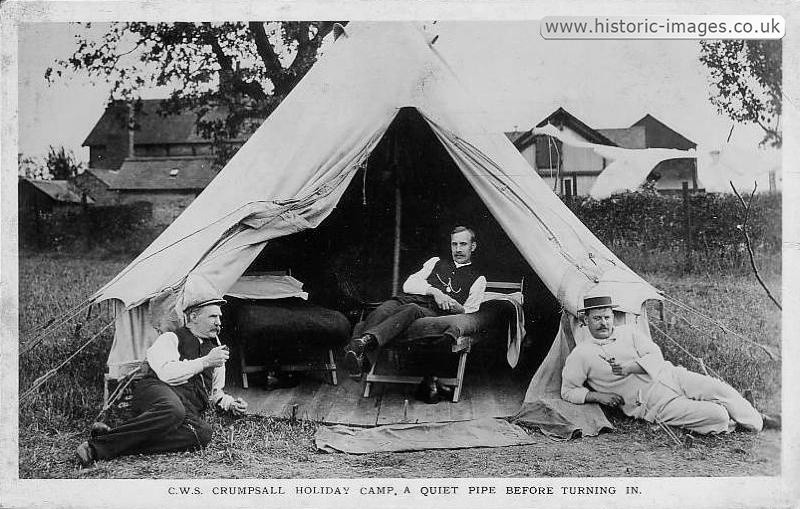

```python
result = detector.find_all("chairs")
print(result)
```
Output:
[222,265,526,402]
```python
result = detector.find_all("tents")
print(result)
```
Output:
[87,21,667,441]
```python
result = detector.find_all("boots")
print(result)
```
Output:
[253,358,297,390]
[425,375,453,403]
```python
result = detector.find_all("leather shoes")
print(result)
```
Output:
[343,334,377,356]
[344,350,370,382]
[91,422,111,437]
[74,441,97,467]
[762,413,779,428]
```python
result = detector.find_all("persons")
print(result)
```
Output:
[561,297,781,435]
[76,304,248,466]
[345,226,486,382]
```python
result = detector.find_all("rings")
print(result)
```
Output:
[613,401,616,405]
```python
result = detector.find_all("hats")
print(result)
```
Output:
[182,273,228,312]
[577,296,619,312]
